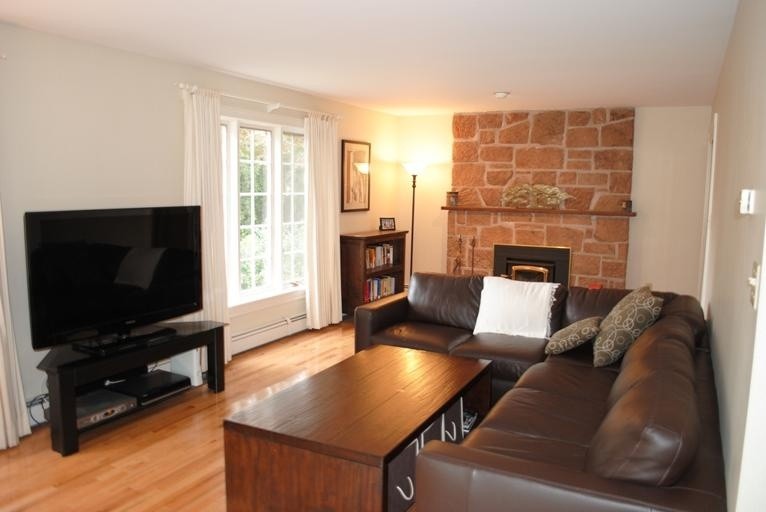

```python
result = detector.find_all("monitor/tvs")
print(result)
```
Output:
[24,205,203,355]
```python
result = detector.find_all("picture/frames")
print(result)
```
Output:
[341,140,371,211]
[379,218,395,230]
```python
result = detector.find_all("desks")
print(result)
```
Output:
[37,323,225,457]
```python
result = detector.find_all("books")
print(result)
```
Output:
[363,243,399,304]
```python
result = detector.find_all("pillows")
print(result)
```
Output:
[544,284,665,366]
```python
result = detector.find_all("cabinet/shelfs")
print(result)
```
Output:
[340,230,409,316]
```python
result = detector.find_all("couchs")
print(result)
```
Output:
[355,272,725,512]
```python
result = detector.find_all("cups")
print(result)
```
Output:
[446,191,459,206]
